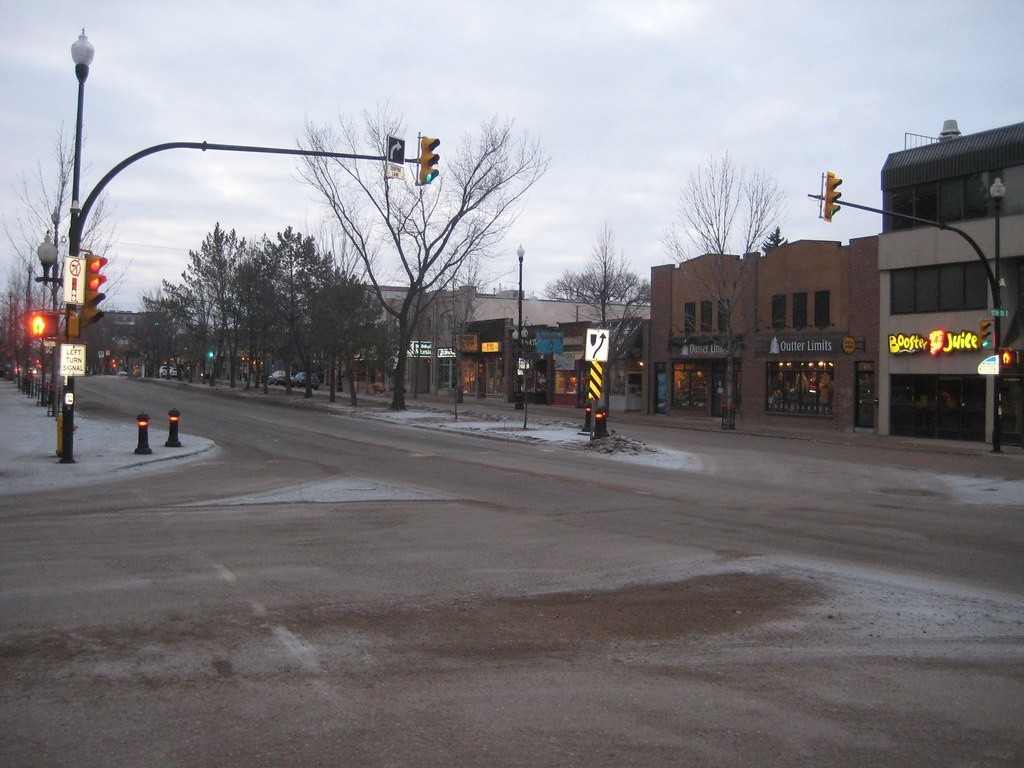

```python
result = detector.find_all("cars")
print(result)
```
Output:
[29,367,37,375]
[290,372,320,390]
[170,368,183,377]
[268,371,295,387]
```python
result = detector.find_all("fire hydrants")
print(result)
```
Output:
[55,415,81,459]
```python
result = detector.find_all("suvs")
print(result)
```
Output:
[159,366,173,377]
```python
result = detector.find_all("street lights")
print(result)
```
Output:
[512,243,529,408]
[33,29,95,465]
[989,177,1010,455]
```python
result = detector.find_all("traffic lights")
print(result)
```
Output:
[67,316,81,338]
[417,134,440,186]
[820,172,842,222]
[30,311,61,337]
[1000,347,1015,367]
[84,253,109,328]
